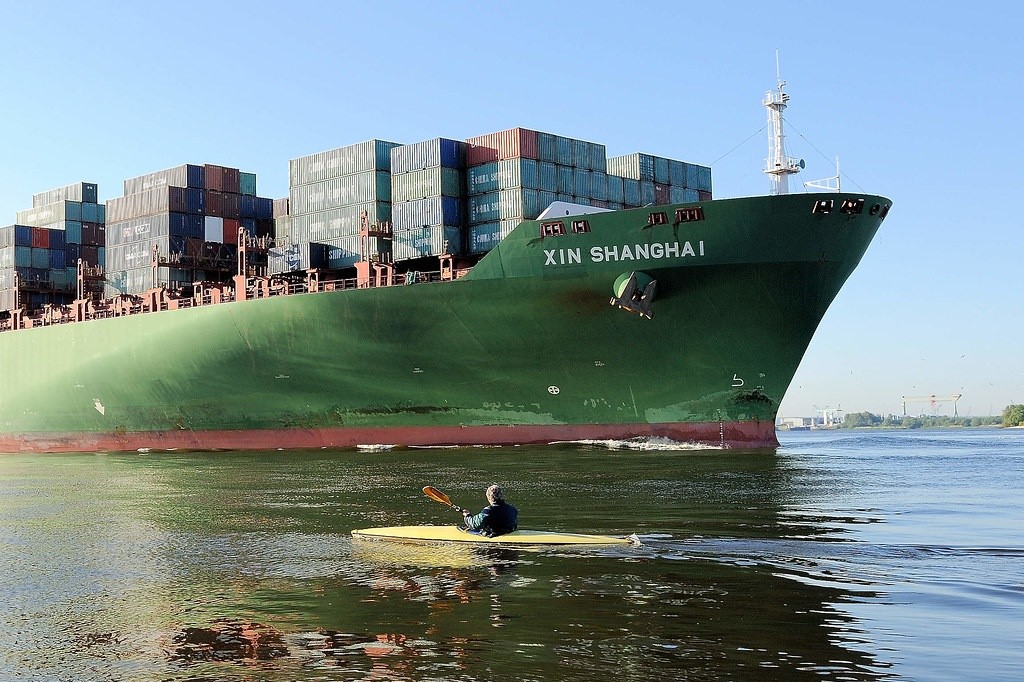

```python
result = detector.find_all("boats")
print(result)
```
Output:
[351,524,636,549]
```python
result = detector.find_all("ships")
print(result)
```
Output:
[0,45,893,454]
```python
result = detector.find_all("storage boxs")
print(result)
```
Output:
[0,126,714,309]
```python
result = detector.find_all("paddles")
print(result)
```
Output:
[422,485,473,519]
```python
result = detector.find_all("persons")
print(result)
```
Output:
[463,484,518,537]
